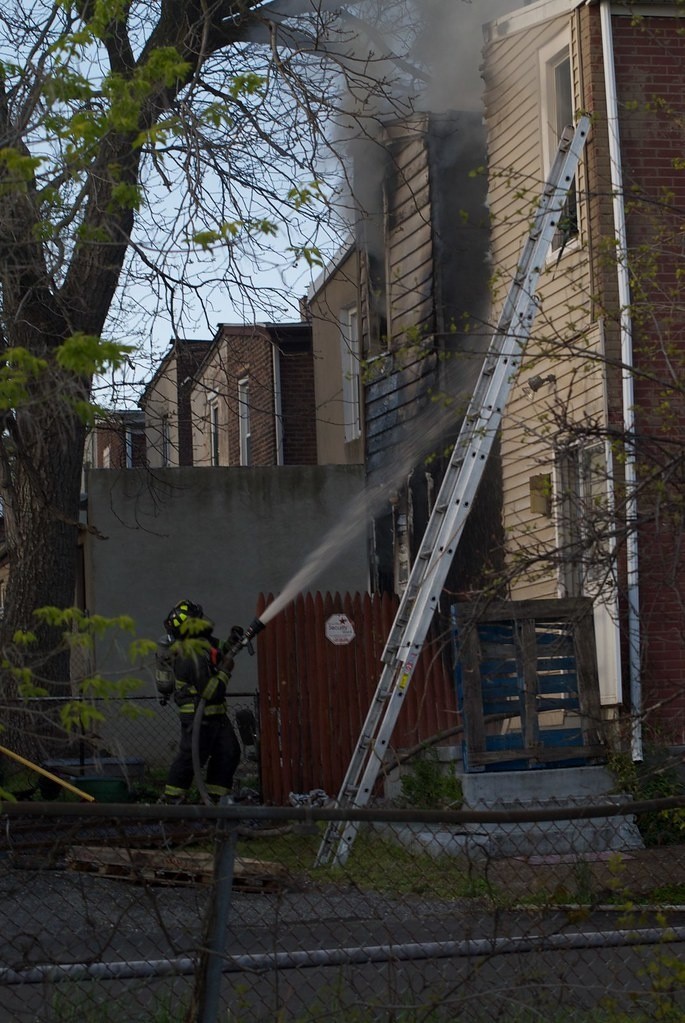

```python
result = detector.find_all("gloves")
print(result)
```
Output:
[229,625,245,642]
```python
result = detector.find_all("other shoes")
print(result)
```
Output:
[156,793,186,806]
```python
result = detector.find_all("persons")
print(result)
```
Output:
[156,599,241,837]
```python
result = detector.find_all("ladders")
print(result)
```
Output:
[310,102,597,876]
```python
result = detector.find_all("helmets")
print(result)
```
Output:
[165,599,204,640]
